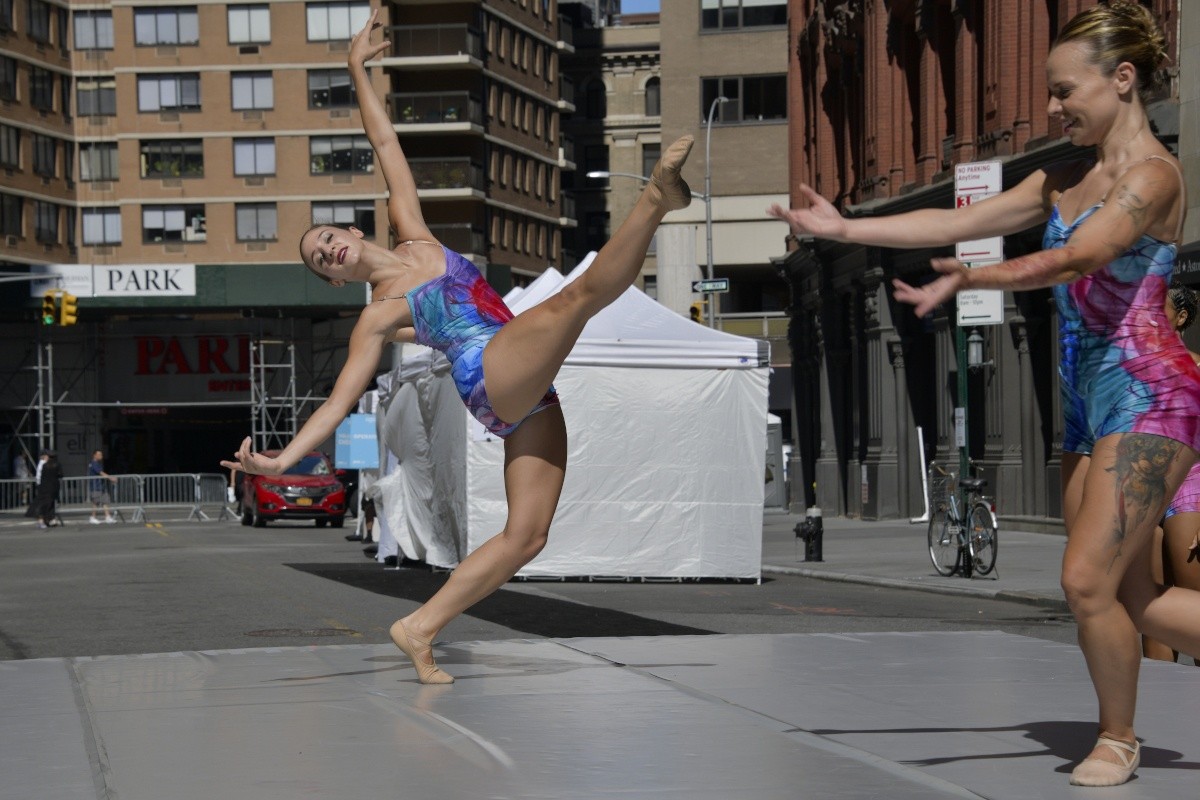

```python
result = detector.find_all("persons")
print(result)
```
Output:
[220,10,697,688]
[769,2,1199,788]
[87,450,118,525]
[14,449,68,528]
[1138,285,1200,668]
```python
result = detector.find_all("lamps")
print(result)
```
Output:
[967,330,993,368]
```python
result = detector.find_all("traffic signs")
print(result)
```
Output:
[691,279,729,296]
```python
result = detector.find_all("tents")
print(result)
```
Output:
[354,249,771,587]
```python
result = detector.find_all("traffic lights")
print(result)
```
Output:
[41,289,58,326]
[60,289,78,327]
[690,301,704,326]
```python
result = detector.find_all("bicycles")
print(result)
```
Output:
[927,457,999,580]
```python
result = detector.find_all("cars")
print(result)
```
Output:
[237,449,346,527]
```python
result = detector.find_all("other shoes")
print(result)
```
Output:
[48,518,58,526]
[89,517,101,525]
[105,516,117,524]
[36,518,47,529]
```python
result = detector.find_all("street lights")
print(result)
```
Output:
[585,95,730,331]
